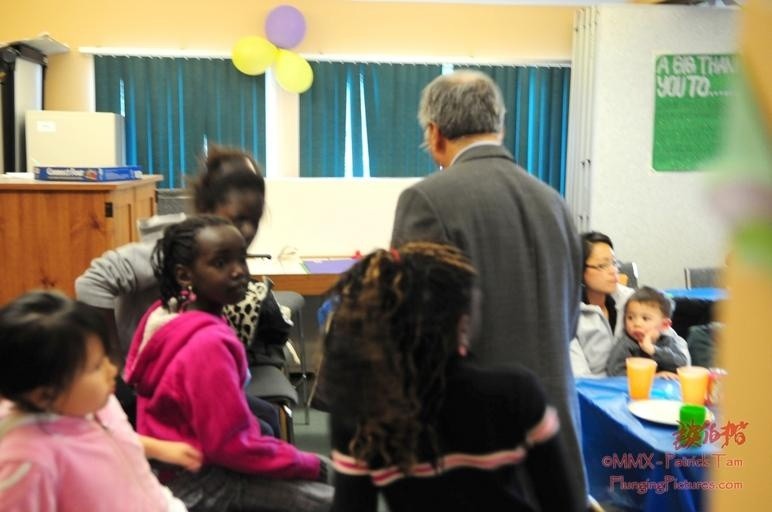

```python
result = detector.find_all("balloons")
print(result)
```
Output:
[230,5,315,94]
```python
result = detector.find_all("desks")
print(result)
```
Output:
[655,287,729,332]
[246,256,365,298]
[572,372,723,511]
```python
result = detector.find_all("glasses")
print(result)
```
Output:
[583,260,621,271]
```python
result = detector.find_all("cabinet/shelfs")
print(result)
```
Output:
[1,167,164,316]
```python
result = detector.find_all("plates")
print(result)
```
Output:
[626,400,715,427]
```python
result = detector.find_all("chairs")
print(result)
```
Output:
[618,260,640,293]
[682,265,725,289]
[246,362,299,448]
[270,290,312,428]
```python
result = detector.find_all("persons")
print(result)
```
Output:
[75,143,278,436]
[0,290,188,511]
[606,285,691,374]
[320,242,589,512]
[121,211,335,512]
[391,71,591,510]
[569,230,692,380]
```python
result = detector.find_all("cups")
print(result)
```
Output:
[626,357,657,402]
[706,368,727,408]
[678,405,707,450]
[617,274,629,286]
[675,366,707,406]
